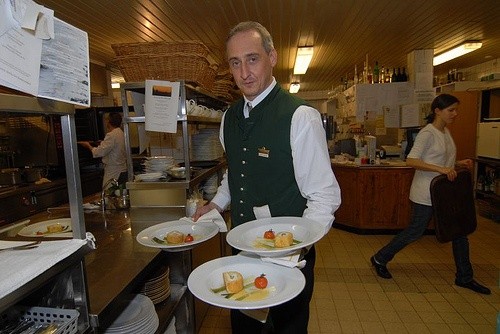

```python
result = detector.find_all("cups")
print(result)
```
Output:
[187,100,223,119]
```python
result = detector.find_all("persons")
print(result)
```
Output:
[191,21,341,334]
[370,93,492,295]
[81,109,128,190]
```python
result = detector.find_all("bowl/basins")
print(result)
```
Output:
[110,189,131,209]
[201,169,219,199]
[169,167,192,178]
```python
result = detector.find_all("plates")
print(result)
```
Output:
[103,266,172,334]
[226,216,327,256]
[137,156,174,182]
[17,217,74,238]
[190,128,225,161]
[136,218,219,252]
[187,255,306,310]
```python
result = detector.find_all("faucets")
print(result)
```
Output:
[98,178,117,211]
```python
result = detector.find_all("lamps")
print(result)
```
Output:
[431,40,482,65]
[289,83,300,93]
[293,46,314,75]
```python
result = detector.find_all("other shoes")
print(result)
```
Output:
[371,256,392,279]
[456,278,490,295]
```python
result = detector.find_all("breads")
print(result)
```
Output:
[222,271,244,293]
[274,232,293,247]
[167,231,183,244]
[47,224,62,232]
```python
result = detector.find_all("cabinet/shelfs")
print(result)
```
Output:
[0,78,228,334]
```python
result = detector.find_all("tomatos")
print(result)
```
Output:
[264,229,274,239]
[255,273,267,289]
[36,231,43,235]
[185,233,193,243]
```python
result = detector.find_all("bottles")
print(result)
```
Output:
[327,60,409,99]
